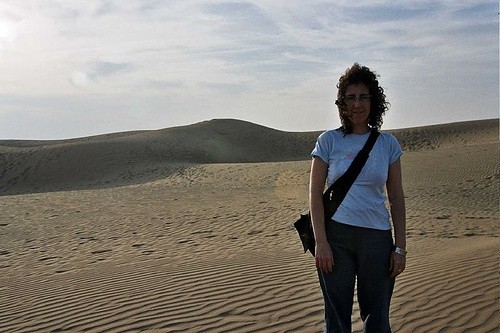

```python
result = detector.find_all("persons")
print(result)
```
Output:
[309,62,408,333]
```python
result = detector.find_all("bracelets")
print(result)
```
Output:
[393,245,408,256]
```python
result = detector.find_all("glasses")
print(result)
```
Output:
[343,94,370,103]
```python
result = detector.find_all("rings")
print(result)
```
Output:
[397,268,402,273]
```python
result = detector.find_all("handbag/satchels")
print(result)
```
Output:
[294,132,380,256]
